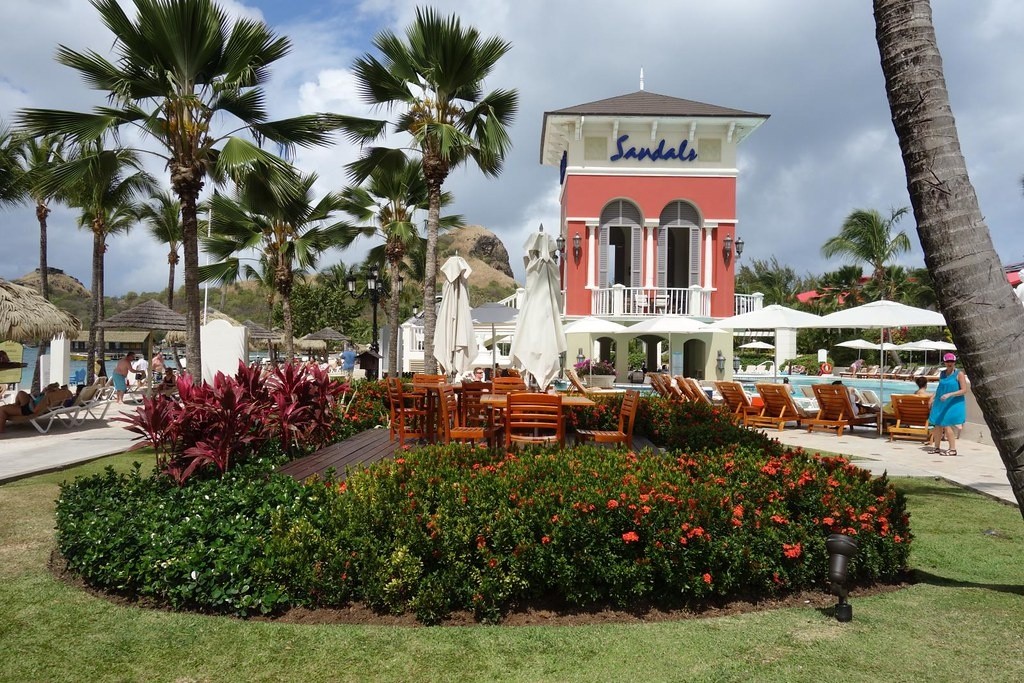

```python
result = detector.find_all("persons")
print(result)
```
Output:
[0,345,357,434]
[473,353,968,456]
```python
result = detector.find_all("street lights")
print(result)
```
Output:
[347,262,380,381]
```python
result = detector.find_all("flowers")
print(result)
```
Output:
[574,359,619,376]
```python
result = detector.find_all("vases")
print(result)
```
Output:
[583,374,617,389]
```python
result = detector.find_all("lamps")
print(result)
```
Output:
[556,233,565,253]
[733,353,741,373]
[735,237,745,258]
[717,351,726,373]
[723,233,733,258]
[826,534,857,604]
[573,232,581,256]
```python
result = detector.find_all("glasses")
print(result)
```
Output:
[945,360,956,363]
[476,372,483,375]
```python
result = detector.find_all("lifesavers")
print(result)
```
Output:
[821,363,832,374]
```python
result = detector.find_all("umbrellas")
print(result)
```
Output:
[0,268,350,400]
[432,223,957,391]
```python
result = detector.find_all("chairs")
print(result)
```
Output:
[748,382,819,432]
[801,384,879,436]
[634,294,670,314]
[386,369,640,453]
[839,364,947,383]
[713,381,763,426]
[888,394,943,441]
[2,379,165,434]
[647,373,726,413]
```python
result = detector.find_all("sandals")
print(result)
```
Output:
[940,449,957,456]
[927,448,941,454]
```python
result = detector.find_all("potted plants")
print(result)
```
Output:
[629,354,645,381]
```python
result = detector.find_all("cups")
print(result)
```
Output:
[548,390,555,395]
[126,379,129,386]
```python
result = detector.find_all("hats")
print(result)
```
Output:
[943,353,956,361]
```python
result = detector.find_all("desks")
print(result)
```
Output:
[454,394,595,438]
[875,411,901,436]
